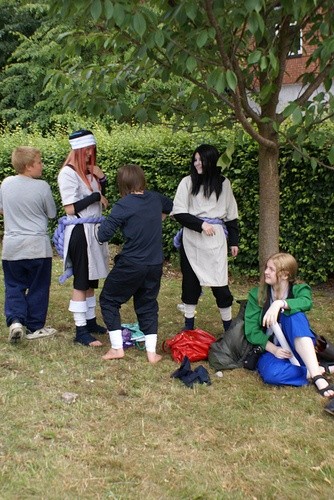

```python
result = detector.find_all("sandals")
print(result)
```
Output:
[311,375,334,398]
[319,363,334,375]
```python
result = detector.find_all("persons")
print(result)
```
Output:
[244,253,334,398]
[51,129,108,346]
[96,164,174,363]
[0,144,58,344]
[169,144,240,332]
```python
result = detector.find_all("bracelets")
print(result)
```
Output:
[280,300,286,313]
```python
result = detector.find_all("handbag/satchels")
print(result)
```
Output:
[121,322,146,349]
[315,334,334,362]
[243,346,264,371]
[162,328,216,363]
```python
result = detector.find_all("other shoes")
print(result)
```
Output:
[75,325,100,347]
[24,326,57,339]
[8,322,23,343]
[86,317,107,335]
